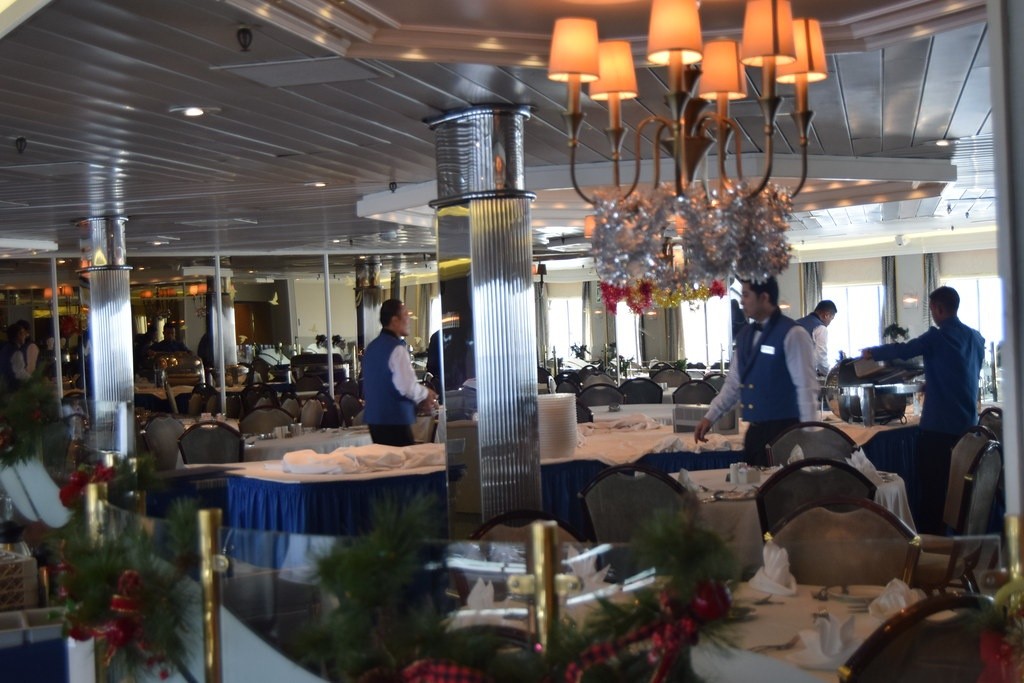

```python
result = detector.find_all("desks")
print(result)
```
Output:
[690,584,957,682]
[243,426,375,462]
[224,403,922,569]
[588,404,676,426]
[666,468,919,569]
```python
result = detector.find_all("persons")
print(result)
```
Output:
[144,323,192,353]
[860,286,985,534]
[0,319,40,391]
[362,299,438,448]
[694,274,817,450]
[795,300,837,375]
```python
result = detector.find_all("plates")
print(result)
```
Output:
[536,392,577,459]
[787,650,840,671]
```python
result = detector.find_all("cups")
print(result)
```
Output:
[260,432,272,440]
[275,425,289,438]
[200,412,212,419]
[290,423,302,437]
[0,495,14,524]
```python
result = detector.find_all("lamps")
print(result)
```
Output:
[547,0,828,204]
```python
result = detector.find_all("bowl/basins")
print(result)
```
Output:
[810,583,885,614]
[0,605,65,649]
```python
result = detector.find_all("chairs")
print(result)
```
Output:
[142,361,1003,683]
